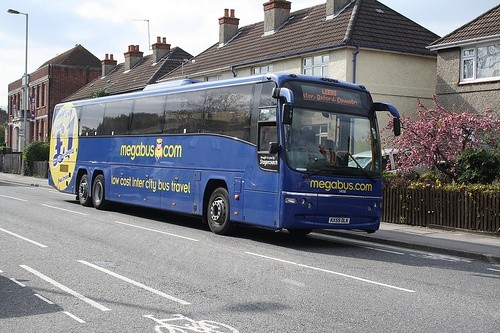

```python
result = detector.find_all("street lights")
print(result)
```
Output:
[7,8,28,177]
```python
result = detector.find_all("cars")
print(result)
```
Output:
[348,148,434,177]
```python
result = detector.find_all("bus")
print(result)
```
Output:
[48,73,401,238]
